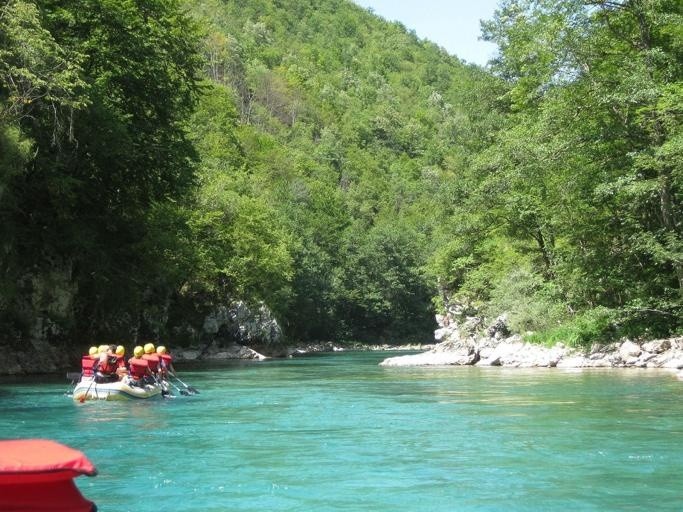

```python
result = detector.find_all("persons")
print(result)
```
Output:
[80,342,177,387]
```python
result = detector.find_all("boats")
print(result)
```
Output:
[72,381,163,401]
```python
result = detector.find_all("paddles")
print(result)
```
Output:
[167,371,200,394]
[165,378,188,396]
[150,374,174,399]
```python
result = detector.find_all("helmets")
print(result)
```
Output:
[134,343,166,356]
[89,345,124,355]
